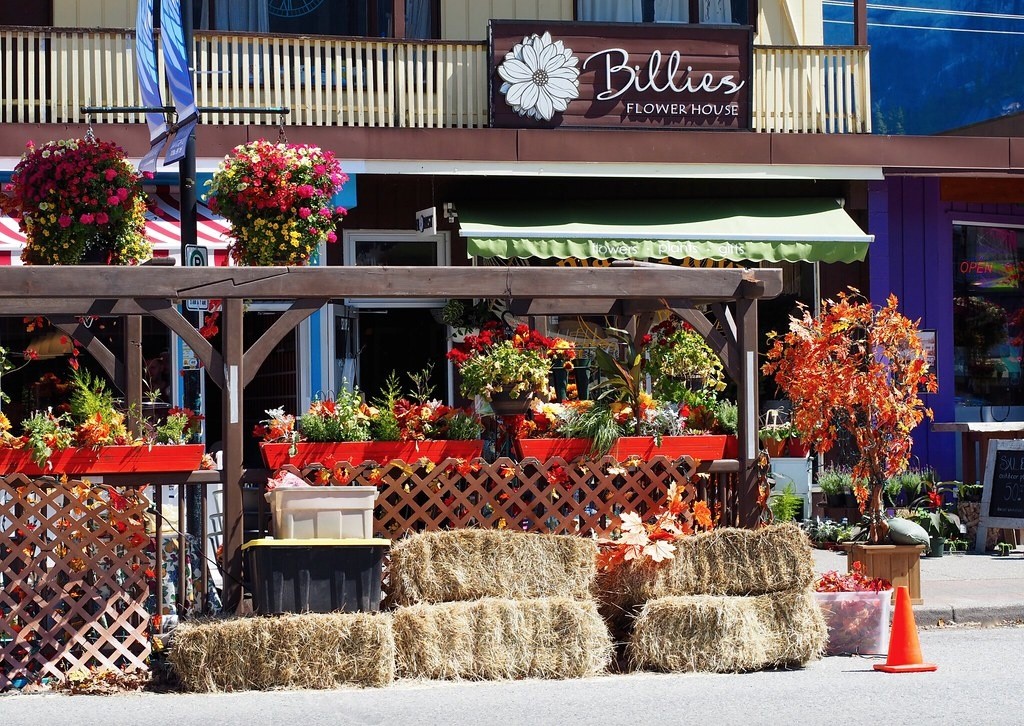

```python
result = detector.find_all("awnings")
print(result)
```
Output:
[0,182,310,266]
[457,200,875,264]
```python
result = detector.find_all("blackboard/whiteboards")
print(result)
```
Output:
[979,439,1024,529]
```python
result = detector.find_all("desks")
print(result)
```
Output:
[930,421,1024,548]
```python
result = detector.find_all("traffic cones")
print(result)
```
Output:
[872,585,939,673]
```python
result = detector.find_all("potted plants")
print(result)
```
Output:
[908,479,964,555]
[714,399,739,459]
[957,486,1000,552]
[759,283,943,606]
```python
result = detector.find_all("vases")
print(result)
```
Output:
[670,373,704,392]
[0,443,204,475]
[489,384,533,415]
[258,439,484,470]
[515,434,727,464]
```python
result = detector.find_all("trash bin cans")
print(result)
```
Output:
[240,538,392,618]
[264,485,380,538]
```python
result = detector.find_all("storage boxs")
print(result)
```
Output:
[263,486,378,539]
[241,538,391,614]
[813,589,895,659]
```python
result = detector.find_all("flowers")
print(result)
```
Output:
[493,326,719,464]
[927,491,944,510]
[4,139,154,266]
[254,358,488,457]
[446,318,576,403]
[201,140,347,266]
[640,312,729,409]
[0,347,205,470]
